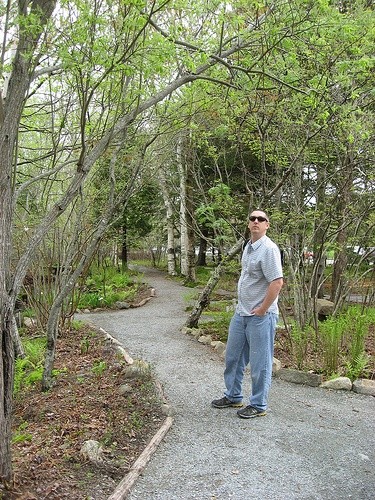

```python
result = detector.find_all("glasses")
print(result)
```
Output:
[249,216,268,222]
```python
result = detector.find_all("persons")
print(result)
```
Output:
[211,209,285,419]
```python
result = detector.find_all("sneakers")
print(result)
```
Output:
[237,404,266,419]
[211,396,243,409]
